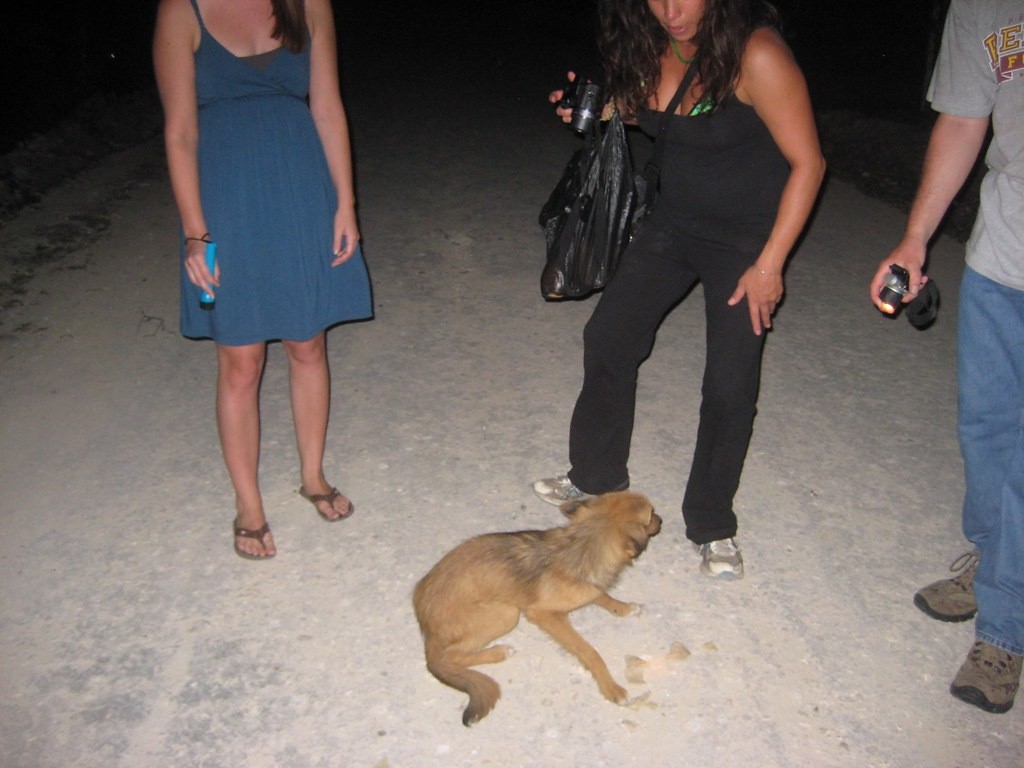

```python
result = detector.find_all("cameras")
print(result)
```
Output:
[560,70,604,133]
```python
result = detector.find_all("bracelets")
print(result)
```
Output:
[185,233,212,245]
[754,263,783,276]
[600,95,615,121]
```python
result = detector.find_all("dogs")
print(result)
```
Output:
[413,493,663,727]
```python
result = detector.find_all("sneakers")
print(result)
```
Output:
[950,638,1023,714]
[531,475,598,507]
[914,551,978,623]
[692,537,743,581]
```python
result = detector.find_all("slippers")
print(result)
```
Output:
[300,485,354,523]
[233,518,277,560]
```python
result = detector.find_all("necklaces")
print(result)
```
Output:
[670,37,697,64]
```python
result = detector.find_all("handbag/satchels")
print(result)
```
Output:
[540,111,635,299]
[538,136,655,259]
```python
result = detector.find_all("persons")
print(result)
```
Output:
[533,0,826,581]
[871,0,1024,714]
[155,0,376,560]
